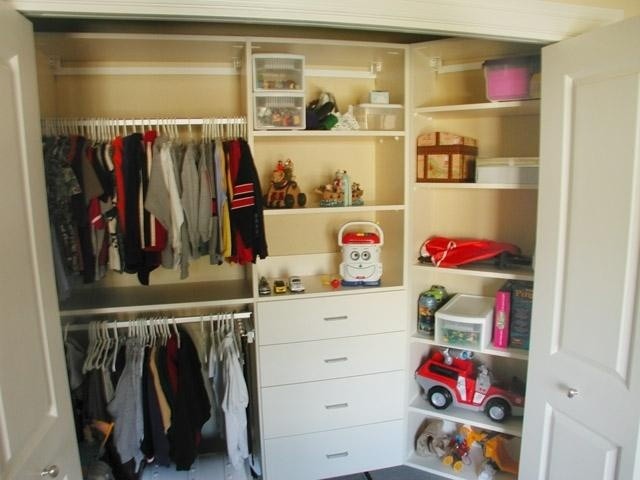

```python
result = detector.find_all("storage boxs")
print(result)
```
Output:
[481,55,543,102]
[350,104,403,132]
[418,132,478,182]
[435,293,497,355]
[252,53,307,130]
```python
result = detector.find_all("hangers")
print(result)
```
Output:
[40,115,250,167]
[62,311,246,374]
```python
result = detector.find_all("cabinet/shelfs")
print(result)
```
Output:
[0,2,640,480]
